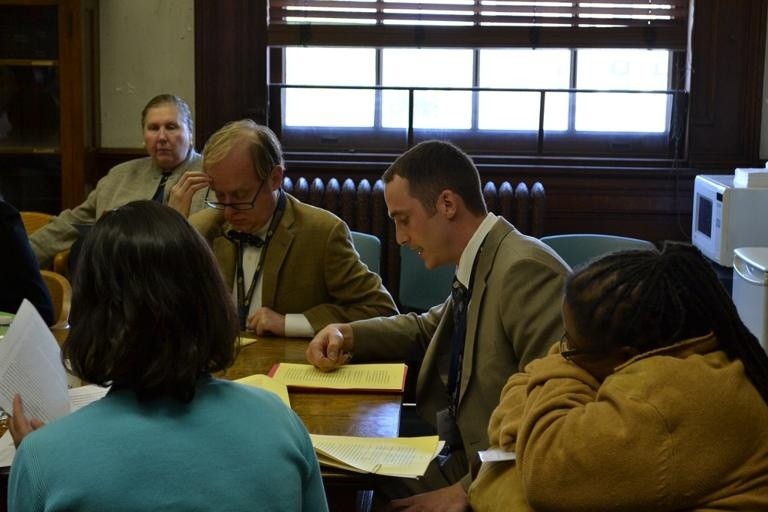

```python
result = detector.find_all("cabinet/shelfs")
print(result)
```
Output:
[0,0,100,216]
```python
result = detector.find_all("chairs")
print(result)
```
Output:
[14,211,668,337]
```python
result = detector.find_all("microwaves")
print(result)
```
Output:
[691,173,768,268]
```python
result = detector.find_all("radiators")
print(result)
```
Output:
[282,176,546,315]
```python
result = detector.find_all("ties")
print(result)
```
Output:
[445,278,469,405]
[152,172,171,204]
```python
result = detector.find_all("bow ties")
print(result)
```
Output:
[226,230,263,248]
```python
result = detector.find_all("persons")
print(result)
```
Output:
[305,139,574,512]
[5,199,333,512]
[25,92,215,269]
[464,236,768,510]
[164,117,400,338]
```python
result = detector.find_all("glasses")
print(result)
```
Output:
[558,330,618,361]
[204,177,266,212]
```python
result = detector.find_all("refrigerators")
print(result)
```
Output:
[731,246,768,354]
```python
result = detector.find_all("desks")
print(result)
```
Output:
[700,254,732,295]
[82,331,403,510]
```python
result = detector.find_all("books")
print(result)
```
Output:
[0,297,113,425]
